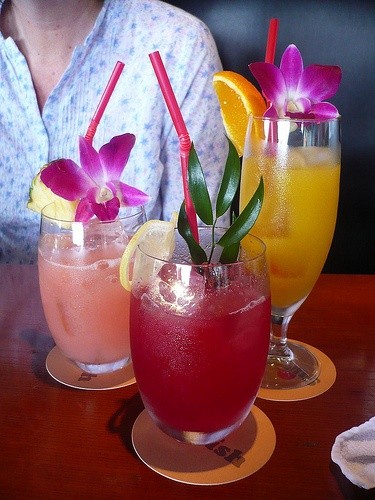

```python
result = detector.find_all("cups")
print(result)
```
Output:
[126,224,272,442]
[36,202,145,374]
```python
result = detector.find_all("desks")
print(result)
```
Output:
[0,264,375,500]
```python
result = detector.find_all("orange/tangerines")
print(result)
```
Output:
[213,70,266,157]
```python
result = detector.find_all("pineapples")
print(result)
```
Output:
[25,162,79,228]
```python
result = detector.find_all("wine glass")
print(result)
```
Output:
[239,116,342,389]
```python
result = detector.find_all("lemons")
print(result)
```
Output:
[119,220,175,292]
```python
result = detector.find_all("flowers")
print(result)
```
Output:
[39,132,152,223]
[248,44,341,127]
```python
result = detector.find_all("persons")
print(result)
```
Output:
[0,0,231,263]
